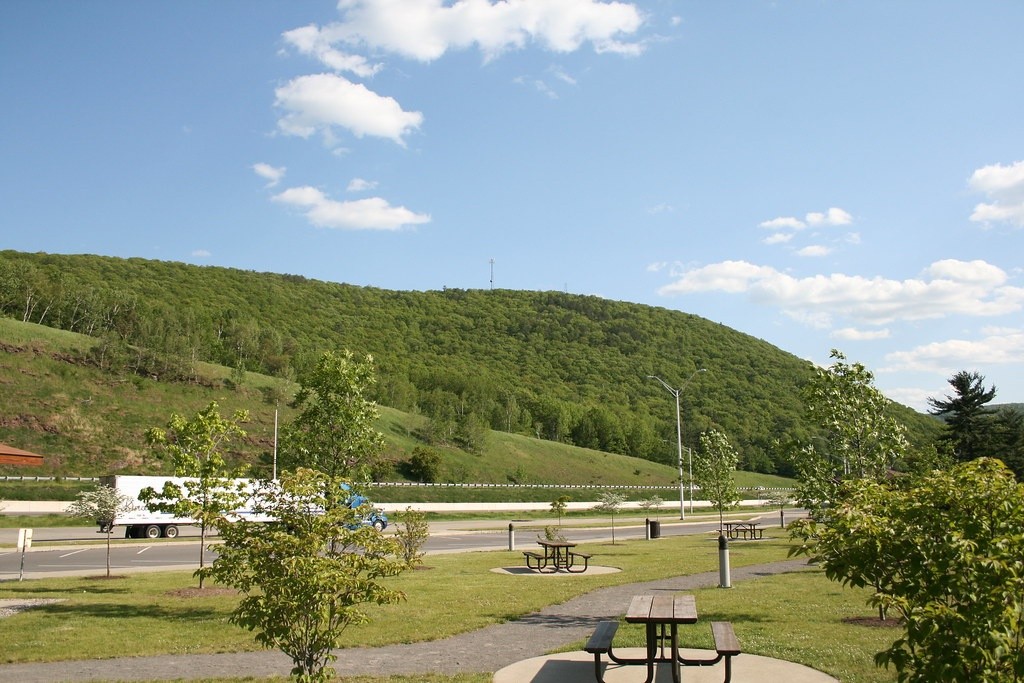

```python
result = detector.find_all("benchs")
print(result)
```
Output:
[554,552,592,572]
[736,529,765,539]
[523,552,555,573]
[716,529,750,540]
[679,622,741,683]
[584,621,647,683]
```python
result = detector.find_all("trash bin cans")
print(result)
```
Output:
[650,520,660,538]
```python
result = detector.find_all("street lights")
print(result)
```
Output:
[648,369,706,520]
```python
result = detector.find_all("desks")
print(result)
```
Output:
[536,541,577,573]
[625,595,698,683]
[723,522,760,540]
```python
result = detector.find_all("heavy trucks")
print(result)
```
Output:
[96,475,388,537]
